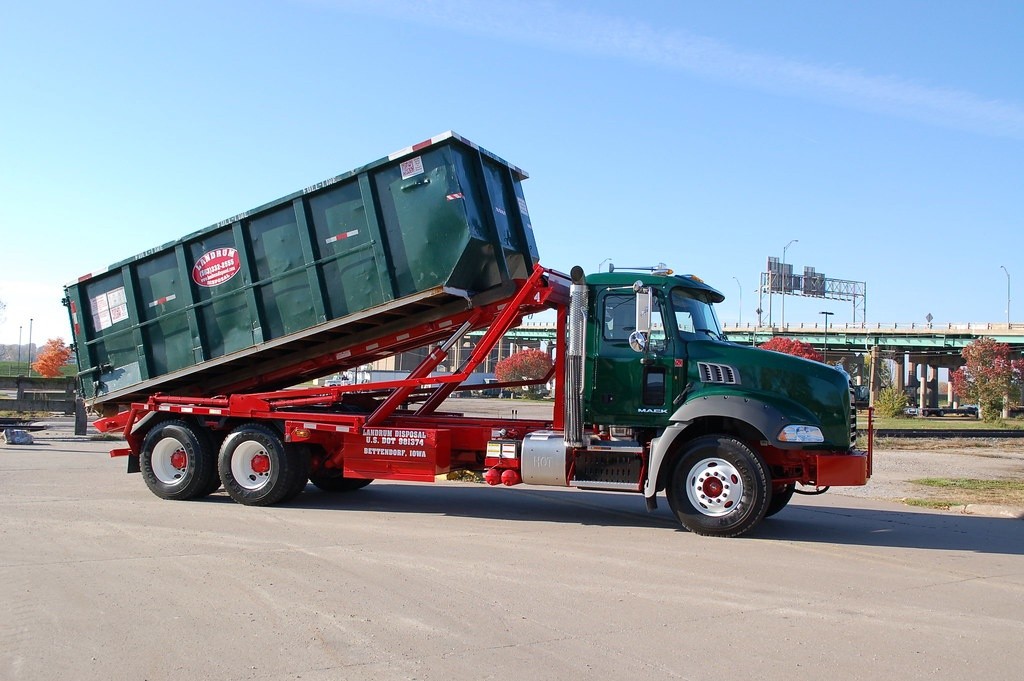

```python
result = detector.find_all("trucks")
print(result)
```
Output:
[922,404,978,418]
[855,385,869,403]
[57,122,878,538]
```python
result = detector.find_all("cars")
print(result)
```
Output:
[902,406,918,415]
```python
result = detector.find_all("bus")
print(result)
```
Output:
[482,377,535,396]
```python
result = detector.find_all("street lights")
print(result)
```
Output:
[1000,265,1010,322]
[732,276,742,328]
[17,326,23,373]
[28,318,33,377]
[598,258,612,273]
[819,310,834,363]
[781,239,799,328]
